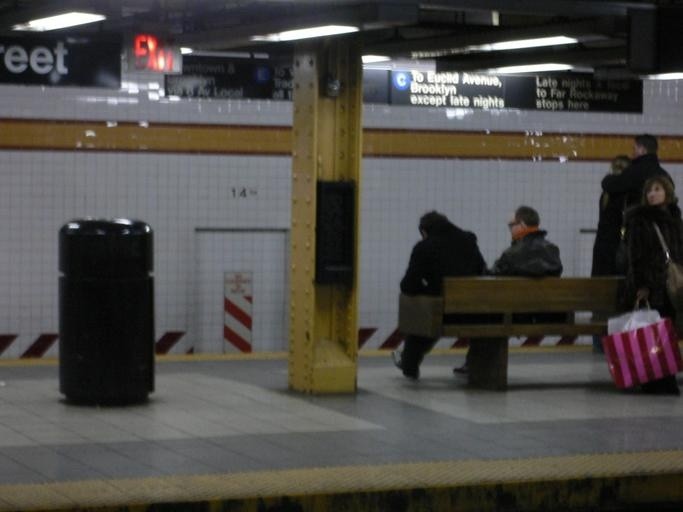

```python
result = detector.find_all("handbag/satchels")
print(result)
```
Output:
[600,295,682,389]
[665,262,683,311]
[615,229,628,266]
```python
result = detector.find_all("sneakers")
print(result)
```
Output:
[391,350,418,378]
[453,366,488,378]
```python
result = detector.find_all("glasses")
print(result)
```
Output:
[508,222,520,229]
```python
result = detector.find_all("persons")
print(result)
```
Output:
[390,208,490,381]
[620,176,683,398]
[584,152,635,355]
[599,134,677,232]
[449,204,564,388]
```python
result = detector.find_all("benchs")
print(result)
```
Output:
[398,275,629,390]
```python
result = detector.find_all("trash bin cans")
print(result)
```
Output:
[55,219,154,405]
